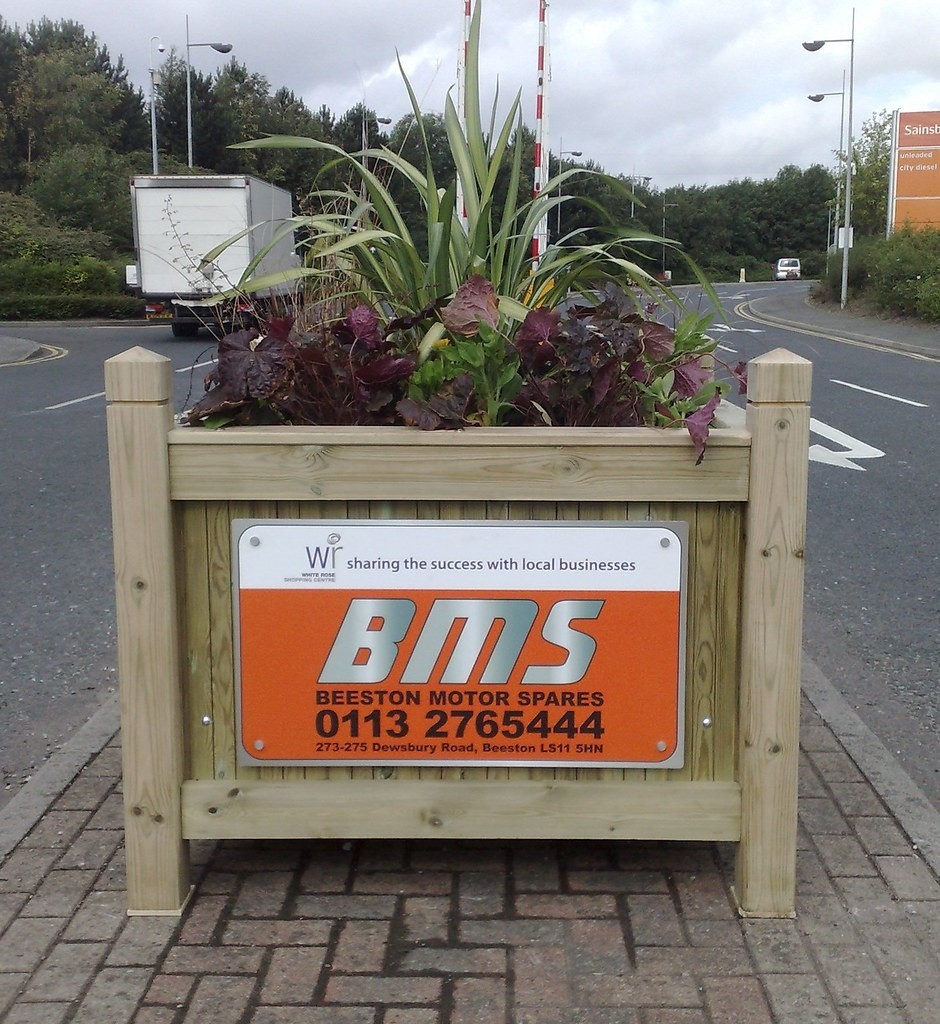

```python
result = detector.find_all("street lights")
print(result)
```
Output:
[184,15,233,172]
[362,118,392,232]
[558,136,581,251]
[630,166,653,286]
[807,70,845,278]
[662,194,678,271]
[803,6,856,308]
[148,35,165,177]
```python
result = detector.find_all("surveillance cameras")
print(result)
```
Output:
[159,45,166,51]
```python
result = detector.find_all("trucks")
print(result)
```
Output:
[126,173,304,340]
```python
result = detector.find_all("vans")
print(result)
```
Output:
[774,259,801,279]
